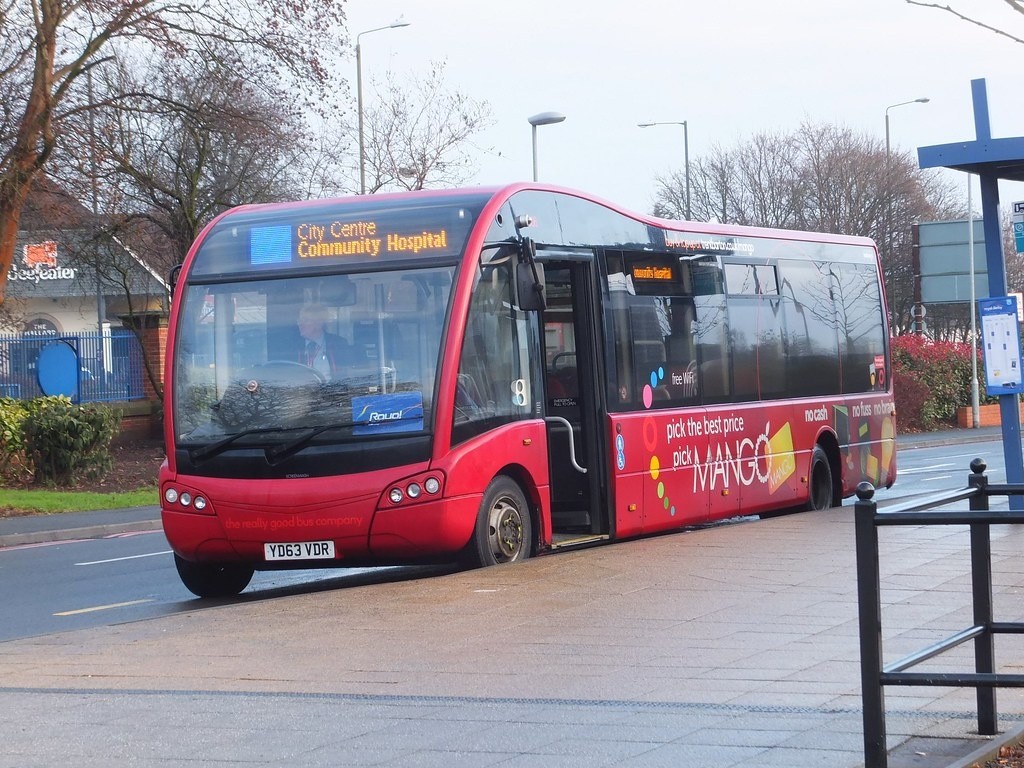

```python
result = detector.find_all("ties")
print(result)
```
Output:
[307,341,316,359]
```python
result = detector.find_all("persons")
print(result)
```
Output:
[291,302,353,382]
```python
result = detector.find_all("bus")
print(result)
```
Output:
[160,185,897,600]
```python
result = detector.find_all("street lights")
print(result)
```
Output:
[529,113,565,184]
[884,96,929,333]
[640,120,692,221]
[356,21,410,190]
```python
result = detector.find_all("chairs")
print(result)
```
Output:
[546,341,723,415]
